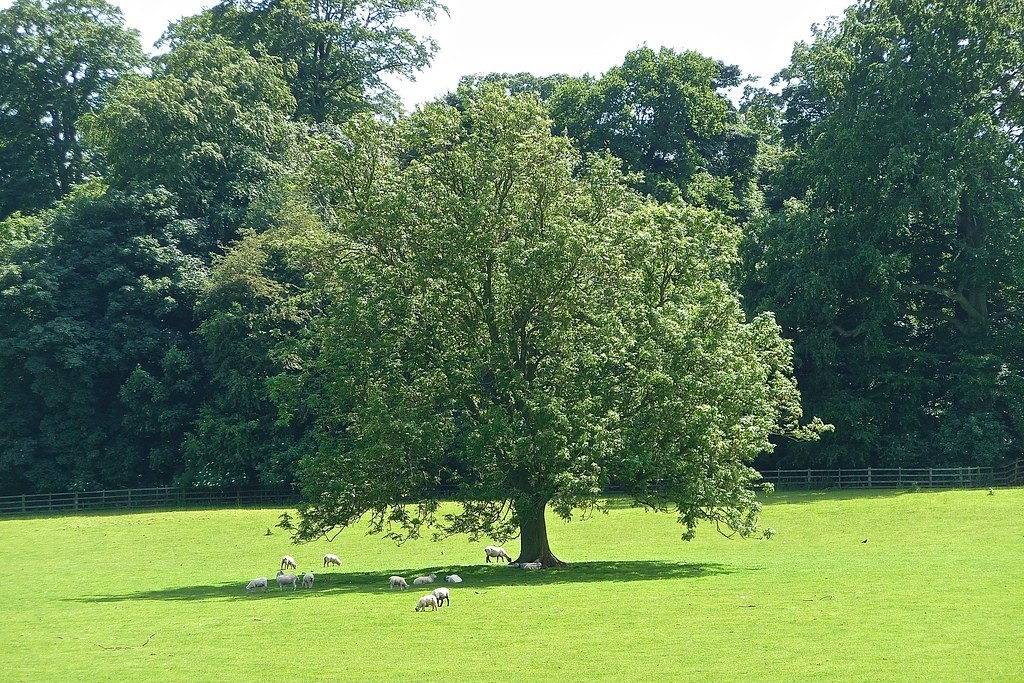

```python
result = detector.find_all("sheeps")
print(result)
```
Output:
[389,576,410,591]
[246,577,271,593]
[413,587,450,612]
[413,573,437,585]
[276,570,299,590]
[445,575,463,583]
[301,572,315,588]
[485,546,512,564]
[281,555,298,570]
[323,554,342,567]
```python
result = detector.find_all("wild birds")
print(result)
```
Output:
[862,539,867,543]
